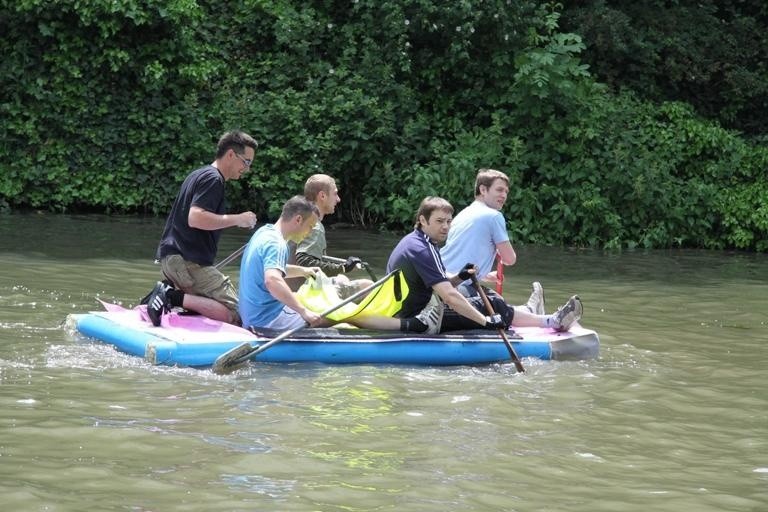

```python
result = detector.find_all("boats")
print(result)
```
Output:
[59,286,604,373]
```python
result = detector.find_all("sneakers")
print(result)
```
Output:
[551,294,583,332]
[415,293,444,336]
[140,281,172,327]
[526,281,545,316]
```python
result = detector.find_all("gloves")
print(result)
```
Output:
[344,256,361,273]
[458,263,474,281]
[483,313,506,330]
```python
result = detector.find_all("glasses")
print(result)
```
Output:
[236,153,252,167]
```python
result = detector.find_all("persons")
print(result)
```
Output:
[236,195,445,337]
[287,173,375,305]
[440,168,517,292]
[386,197,582,333]
[138,130,257,328]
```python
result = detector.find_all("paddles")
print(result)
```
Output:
[140,241,247,304]
[214,269,398,368]
